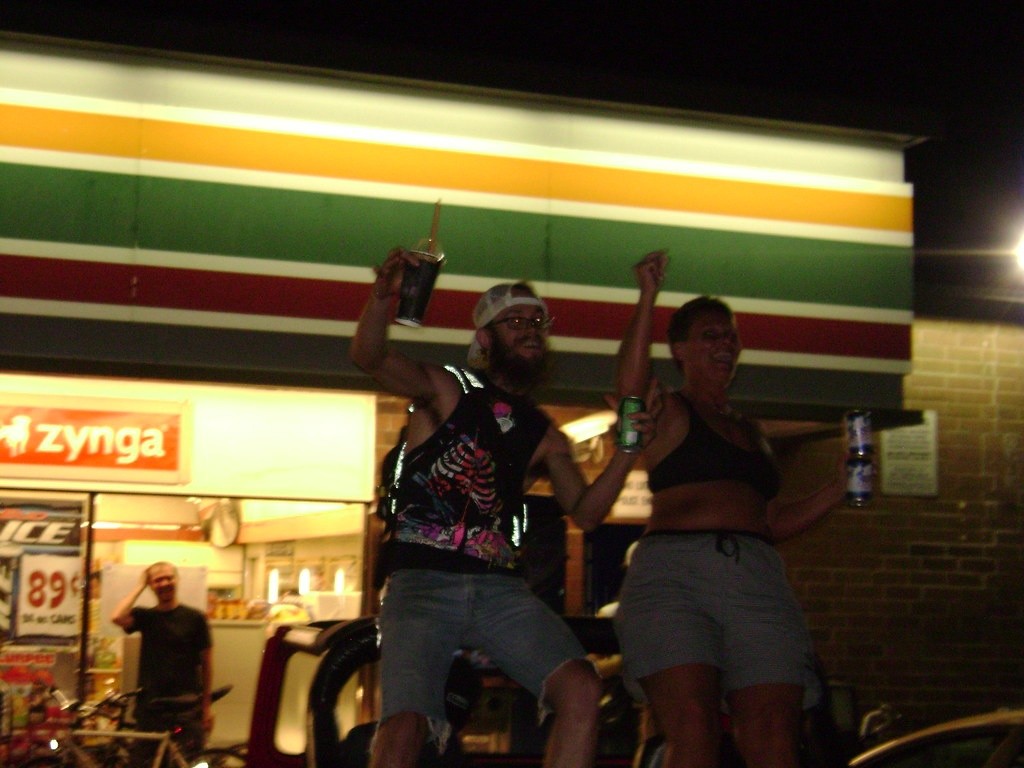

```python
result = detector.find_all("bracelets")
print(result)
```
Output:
[373,286,391,299]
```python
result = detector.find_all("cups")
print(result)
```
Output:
[395,238,446,329]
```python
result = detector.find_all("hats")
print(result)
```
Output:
[467,284,548,370]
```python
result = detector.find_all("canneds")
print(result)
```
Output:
[616,395,646,452]
[840,408,876,508]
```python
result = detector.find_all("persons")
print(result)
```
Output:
[613,253,881,768]
[113,562,214,768]
[349,245,662,768]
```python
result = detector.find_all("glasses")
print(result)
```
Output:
[488,316,543,330]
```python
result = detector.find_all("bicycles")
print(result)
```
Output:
[39,678,249,768]
